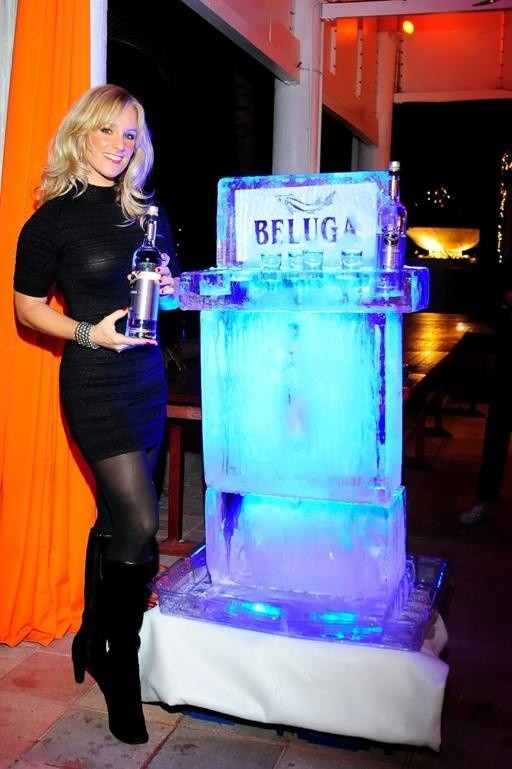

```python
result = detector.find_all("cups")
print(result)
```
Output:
[260,250,362,269]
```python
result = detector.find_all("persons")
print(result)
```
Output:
[459,321,512,525]
[14,85,180,744]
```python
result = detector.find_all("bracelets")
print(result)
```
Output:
[75,321,100,350]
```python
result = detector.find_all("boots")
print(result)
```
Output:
[71,528,159,744]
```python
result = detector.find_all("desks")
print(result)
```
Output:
[156,310,481,556]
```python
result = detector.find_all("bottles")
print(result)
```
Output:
[124,204,161,342]
[379,158,410,269]
